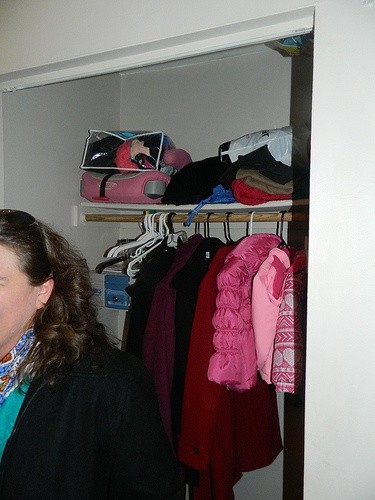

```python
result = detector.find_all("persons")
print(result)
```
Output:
[0,208,196,500]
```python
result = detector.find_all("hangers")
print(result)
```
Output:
[95,208,308,285]
